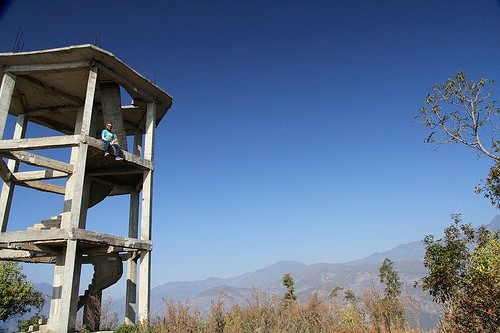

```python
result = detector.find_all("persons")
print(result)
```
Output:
[101,122,123,160]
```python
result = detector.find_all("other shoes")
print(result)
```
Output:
[115,156,123,161]
[104,152,110,156]
